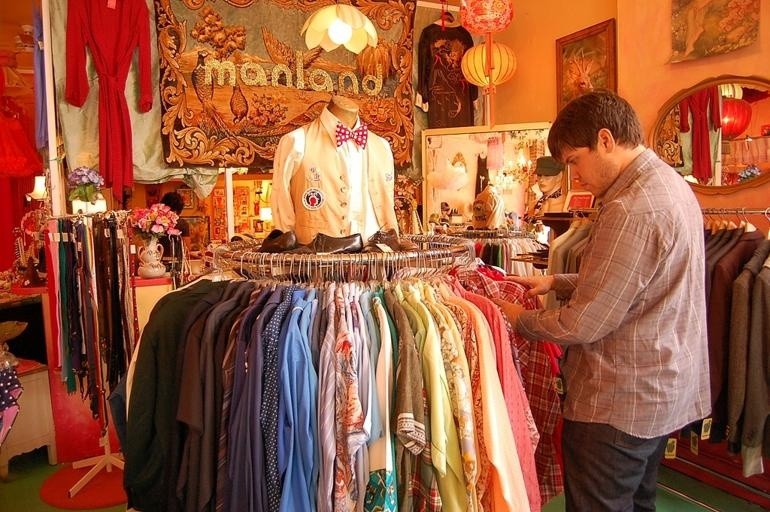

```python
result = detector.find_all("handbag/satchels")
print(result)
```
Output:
[470,186,502,228]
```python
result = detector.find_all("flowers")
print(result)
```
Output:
[127,202,179,239]
[68,166,107,202]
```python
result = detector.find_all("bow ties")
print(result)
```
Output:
[336,121,368,150]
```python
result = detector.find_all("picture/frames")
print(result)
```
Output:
[211,185,251,240]
[177,184,195,210]
[180,215,211,250]
[253,220,264,232]
[553,17,617,120]
[564,187,598,214]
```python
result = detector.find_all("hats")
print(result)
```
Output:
[532,156,565,176]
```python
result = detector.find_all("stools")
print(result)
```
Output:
[1,355,58,482]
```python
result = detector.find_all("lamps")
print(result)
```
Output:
[426,155,468,191]
[254,191,273,223]
[70,197,107,215]
[500,150,532,185]
[299,0,378,56]
[26,173,51,208]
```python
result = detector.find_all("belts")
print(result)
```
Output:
[48,210,142,438]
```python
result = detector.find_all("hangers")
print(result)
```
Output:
[433,0,455,26]
[449,228,526,241]
[567,204,770,240]
[213,234,476,280]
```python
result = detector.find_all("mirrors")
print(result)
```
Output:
[644,67,770,201]
[416,121,570,238]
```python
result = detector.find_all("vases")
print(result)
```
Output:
[138,238,166,277]
[78,187,90,201]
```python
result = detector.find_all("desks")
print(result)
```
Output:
[130,275,174,339]
[1,284,66,463]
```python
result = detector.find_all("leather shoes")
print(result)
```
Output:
[254,228,418,254]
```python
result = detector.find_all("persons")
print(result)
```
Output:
[492,90,712,512]
[155,193,191,272]
[271,97,401,267]
[529,155,567,218]
